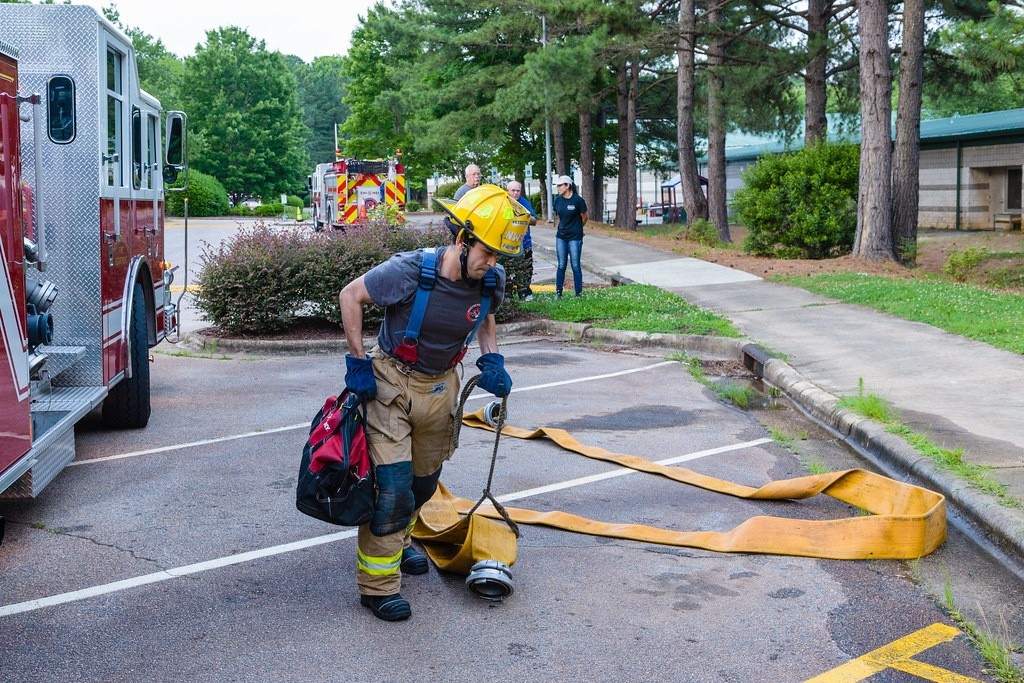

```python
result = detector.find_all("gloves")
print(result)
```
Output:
[345,354,377,400]
[476,353,512,397]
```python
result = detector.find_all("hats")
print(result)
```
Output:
[552,176,572,185]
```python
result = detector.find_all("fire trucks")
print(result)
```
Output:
[308,149,405,234]
[0,0,186,549]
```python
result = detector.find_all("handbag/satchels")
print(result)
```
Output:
[295,386,375,526]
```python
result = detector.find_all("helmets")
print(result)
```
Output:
[431,184,531,257]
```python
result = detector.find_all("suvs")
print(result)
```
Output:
[636,206,673,225]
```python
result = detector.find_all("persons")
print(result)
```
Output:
[453,164,537,302]
[552,176,589,302]
[339,184,531,622]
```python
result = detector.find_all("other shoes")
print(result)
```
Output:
[361,593,412,620]
[525,295,532,301]
[554,295,560,301]
[400,546,429,574]
